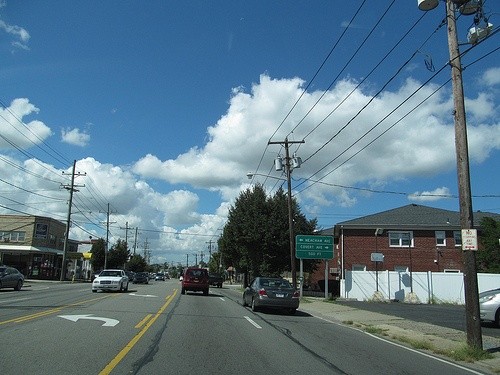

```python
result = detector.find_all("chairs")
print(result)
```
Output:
[261,280,269,286]
[274,281,282,286]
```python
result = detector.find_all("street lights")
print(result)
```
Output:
[246,171,297,288]
[60,210,92,279]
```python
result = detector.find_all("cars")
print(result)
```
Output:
[0,266,25,291]
[479,288,500,324]
[133,271,169,284]
[91,269,130,293]
[243,276,299,314]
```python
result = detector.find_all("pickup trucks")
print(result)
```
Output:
[209,272,223,288]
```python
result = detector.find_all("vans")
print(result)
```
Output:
[181,267,210,296]
